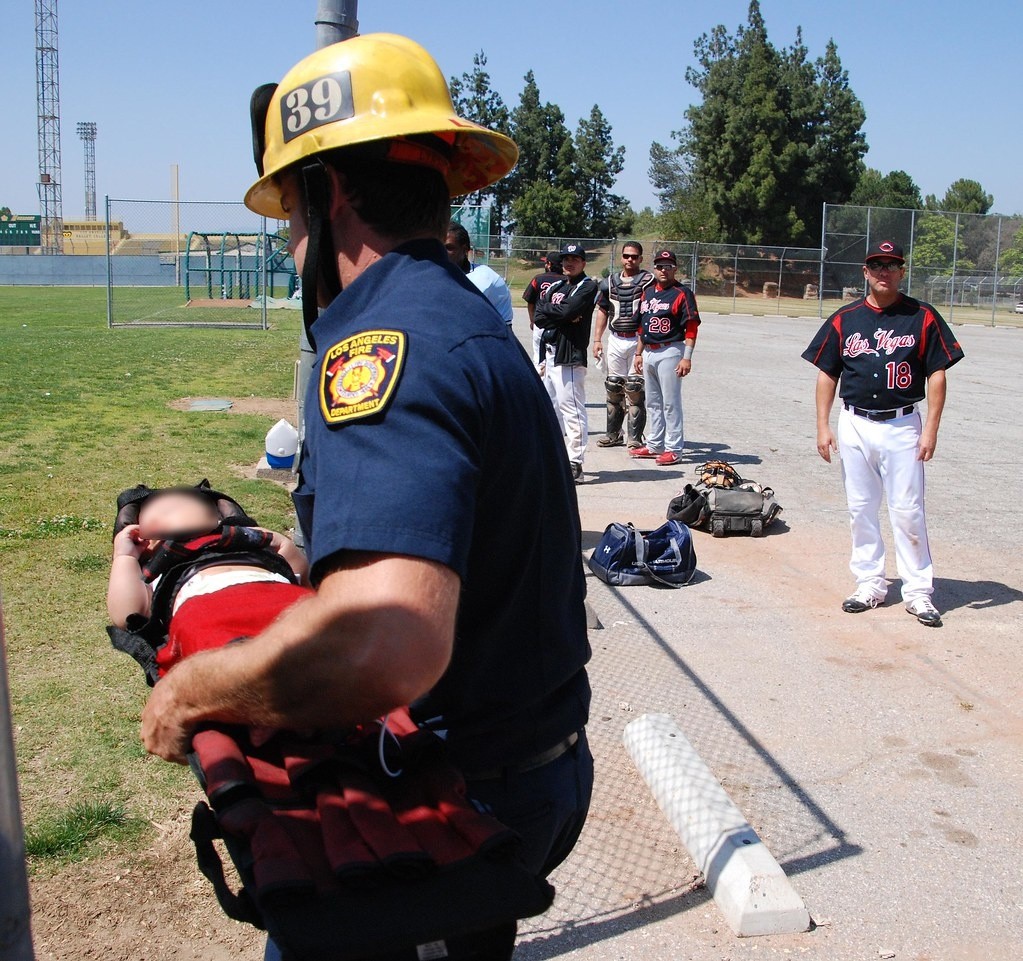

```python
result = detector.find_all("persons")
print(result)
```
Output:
[445,221,513,330]
[105,32,593,961]
[593,240,701,466]
[521,245,598,485]
[801,241,965,627]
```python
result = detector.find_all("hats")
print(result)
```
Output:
[866,239,906,263]
[559,244,585,261]
[540,251,563,268]
[654,250,676,264]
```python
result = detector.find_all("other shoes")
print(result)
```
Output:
[570,460,585,486]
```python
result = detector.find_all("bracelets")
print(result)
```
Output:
[635,353,641,356]
[594,340,601,342]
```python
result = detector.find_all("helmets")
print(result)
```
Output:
[246,34,520,221]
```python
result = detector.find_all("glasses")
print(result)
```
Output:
[866,260,903,272]
[621,253,641,260]
[446,243,462,251]
[654,263,676,271]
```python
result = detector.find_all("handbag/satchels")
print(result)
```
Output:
[589,520,697,589]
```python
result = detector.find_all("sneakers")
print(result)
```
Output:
[842,588,885,613]
[906,597,942,626]
[596,434,623,446]
[627,440,643,448]
[656,451,682,464]
[628,447,665,458]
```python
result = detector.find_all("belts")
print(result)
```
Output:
[502,731,578,779]
[612,331,636,338]
[644,342,671,350]
[844,402,914,421]
